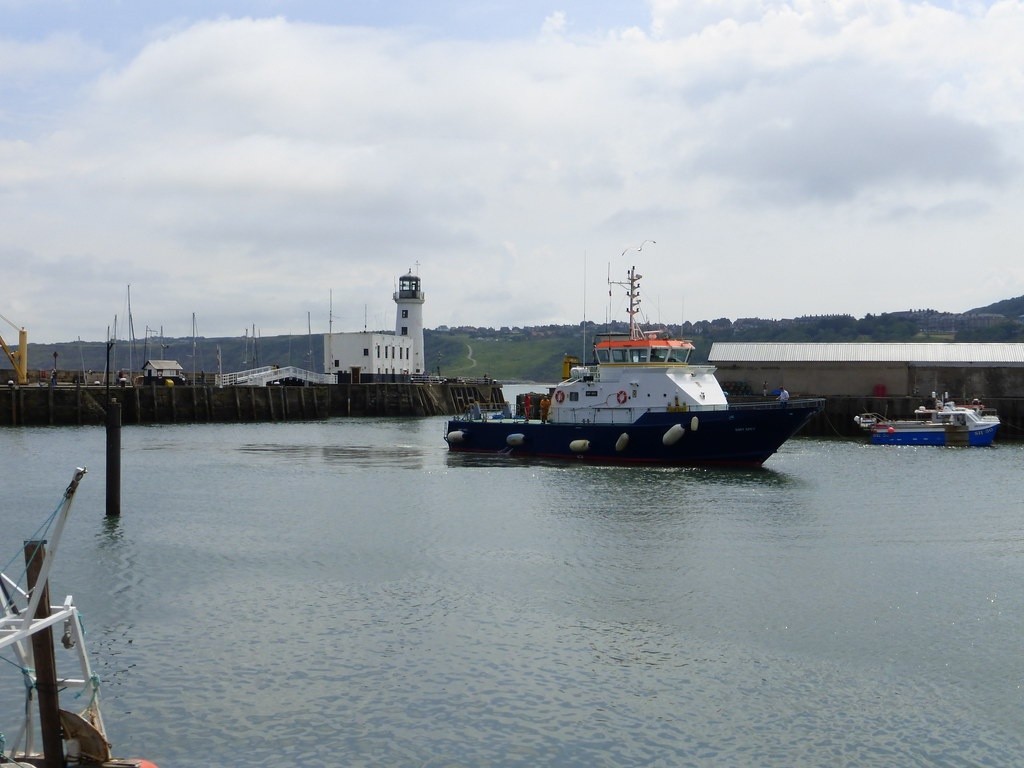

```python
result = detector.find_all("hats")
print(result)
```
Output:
[776,385,784,391]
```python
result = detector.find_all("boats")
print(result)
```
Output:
[853,390,1001,447]
[445,240,828,474]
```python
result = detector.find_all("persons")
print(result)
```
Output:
[540,396,550,423]
[776,387,789,404]
